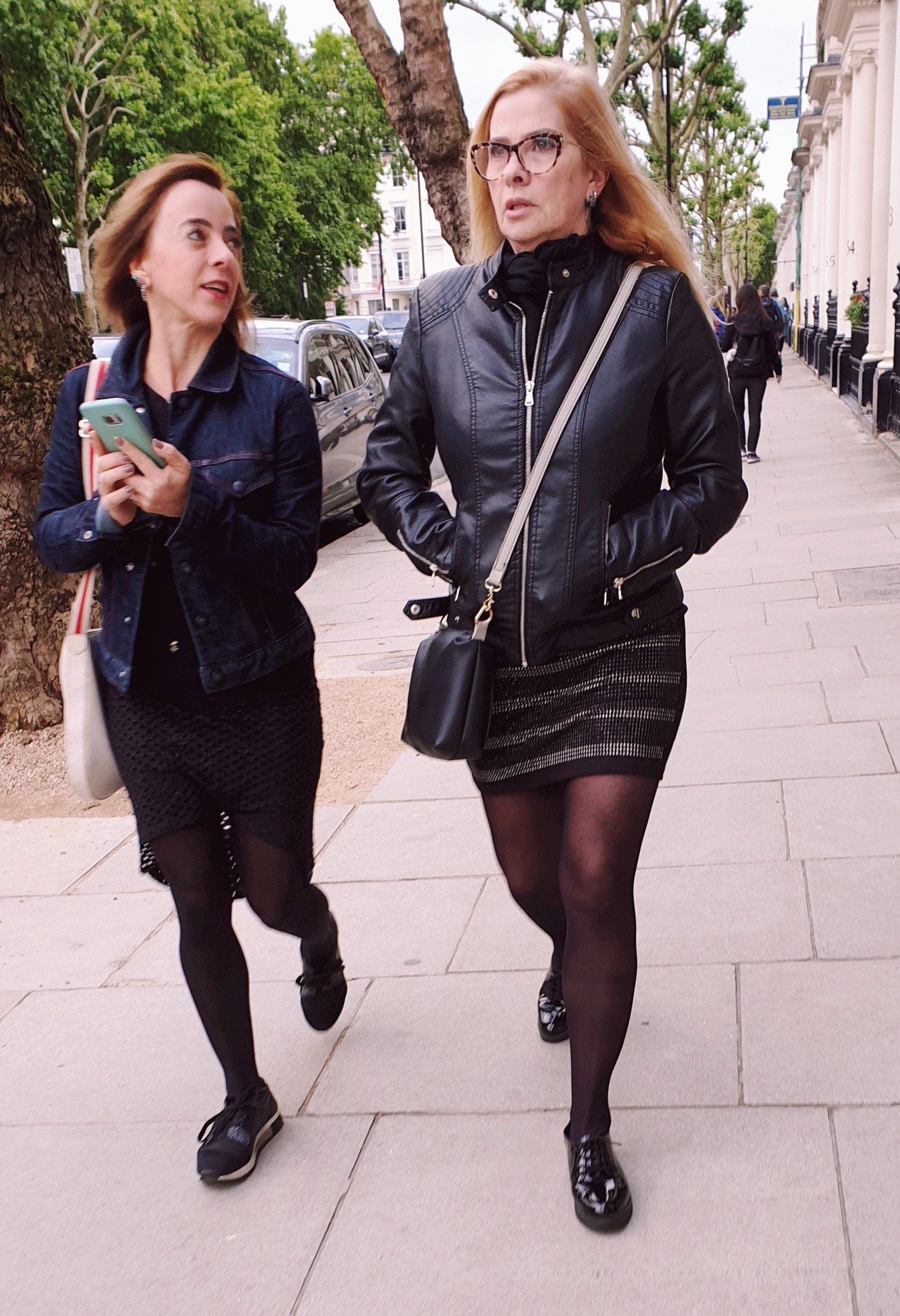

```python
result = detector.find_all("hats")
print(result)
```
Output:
[770,289,778,297]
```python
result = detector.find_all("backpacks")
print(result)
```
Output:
[761,297,776,319]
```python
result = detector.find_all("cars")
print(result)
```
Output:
[328,315,394,373]
[93,316,385,523]
[374,311,409,353]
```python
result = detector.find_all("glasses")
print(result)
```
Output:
[470,133,593,181]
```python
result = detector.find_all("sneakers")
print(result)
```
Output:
[741,451,760,464]
[197,1076,284,1185]
[296,939,348,1030]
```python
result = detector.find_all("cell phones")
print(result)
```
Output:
[80,398,167,473]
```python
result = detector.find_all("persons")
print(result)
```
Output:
[32,152,346,1186]
[708,283,791,464]
[356,57,749,1234]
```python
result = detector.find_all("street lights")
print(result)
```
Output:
[625,34,672,206]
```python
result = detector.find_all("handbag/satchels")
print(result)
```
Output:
[400,626,498,763]
[58,634,124,801]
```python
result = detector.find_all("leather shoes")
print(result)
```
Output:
[538,967,569,1042]
[563,1117,633,1232]
